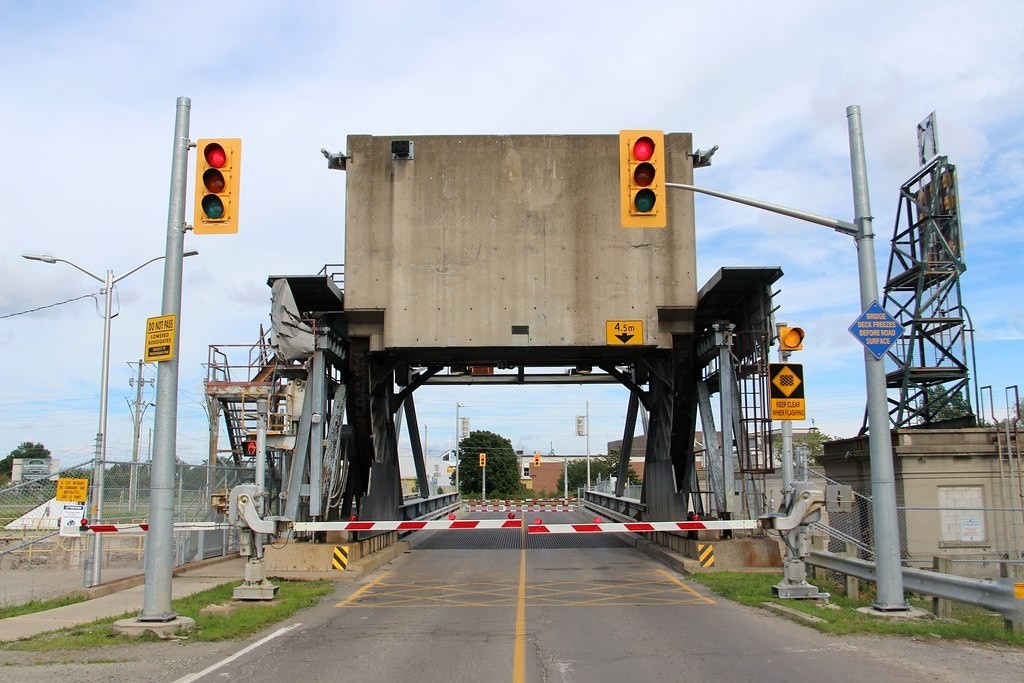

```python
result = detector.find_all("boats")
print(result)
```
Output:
[732,436,796,471]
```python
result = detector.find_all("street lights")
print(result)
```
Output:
[21,250,199,587]
[424,422,428,473]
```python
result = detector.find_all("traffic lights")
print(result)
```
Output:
[778,326,804,351]
[533,453,541,467]
[619,130,667,230]
[479,453,486,467]
[192,138,239,234]
